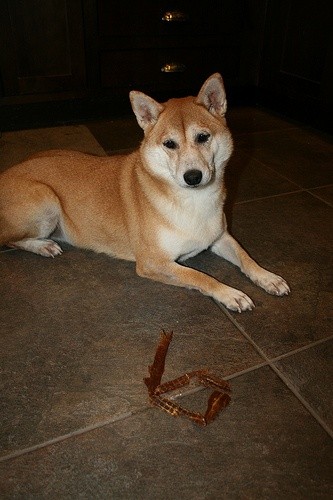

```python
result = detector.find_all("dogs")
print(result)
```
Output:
[1,72,293,314]
